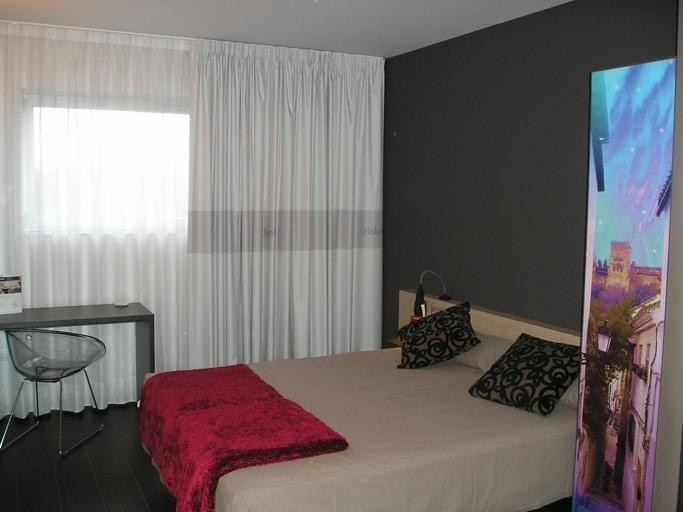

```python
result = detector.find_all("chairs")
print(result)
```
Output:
[0,327,105,456]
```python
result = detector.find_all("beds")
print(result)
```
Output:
[137,288,582,512]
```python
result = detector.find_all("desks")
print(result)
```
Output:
[0,302,155,399]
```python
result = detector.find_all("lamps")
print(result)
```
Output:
[413,270,451,315]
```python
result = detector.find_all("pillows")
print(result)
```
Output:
[395,302,480,368]
[468,333,582,416]
